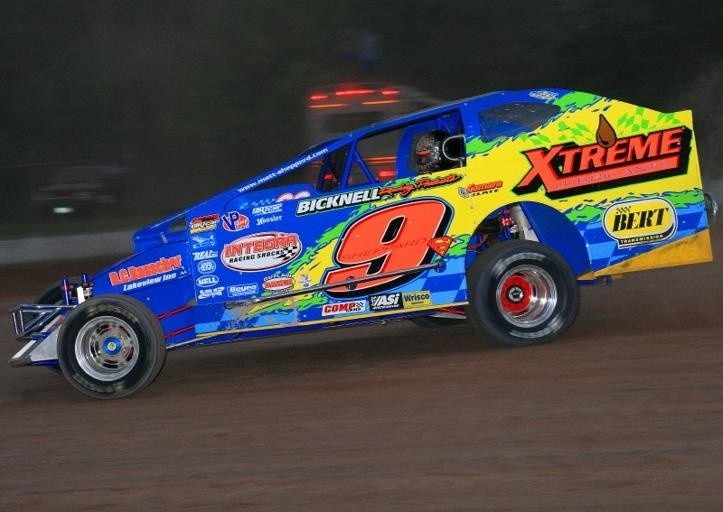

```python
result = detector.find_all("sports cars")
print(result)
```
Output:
[8,83,722,400]
